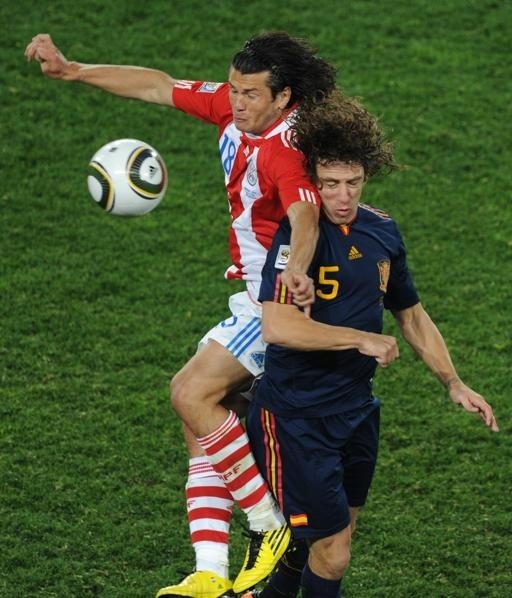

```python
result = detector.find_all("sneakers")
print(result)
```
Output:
[156,570,238,598]
[232,523,296,593]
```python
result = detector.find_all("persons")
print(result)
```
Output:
[22,32,337,598]
[241,96,500,598]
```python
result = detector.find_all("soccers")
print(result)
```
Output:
[88,138,168,217]
